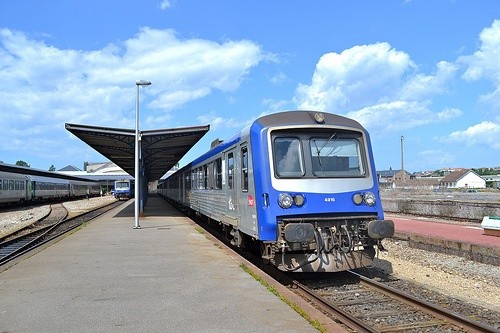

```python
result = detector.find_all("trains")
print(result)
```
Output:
[0,168,104,209]
[113,178,134,201]
[156,109,395,275]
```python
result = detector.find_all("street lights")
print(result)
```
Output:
[134,78,152,231]
